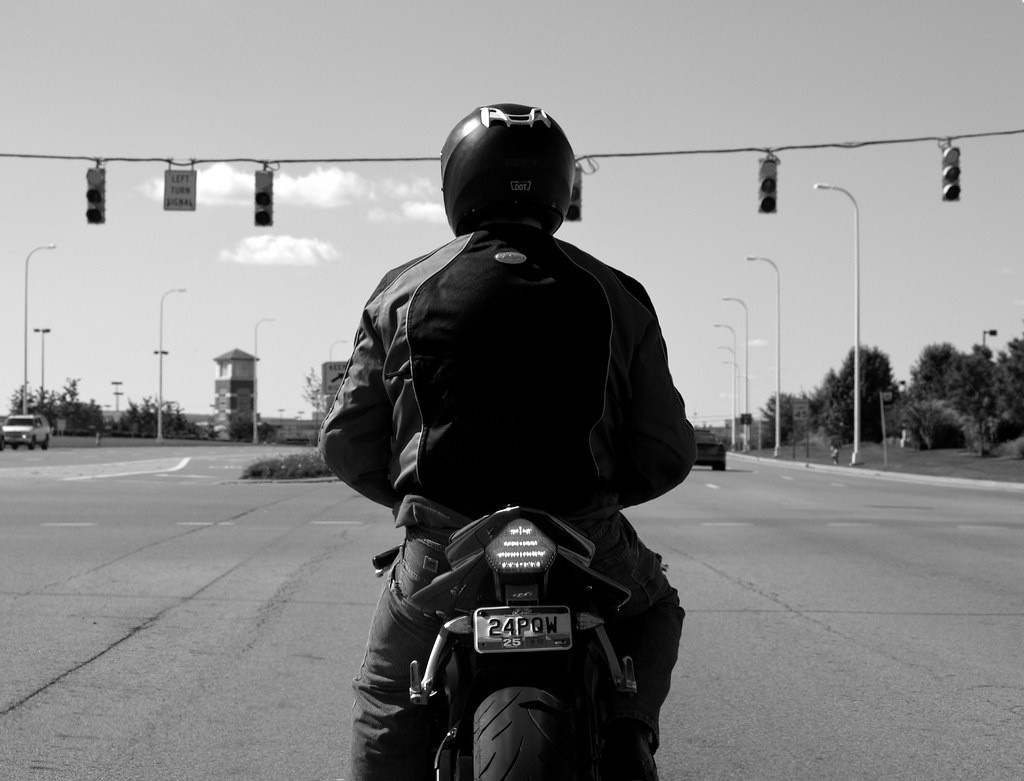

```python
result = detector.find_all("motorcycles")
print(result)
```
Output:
[370,504,639,781]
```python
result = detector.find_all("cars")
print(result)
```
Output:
[692,431,727,471]
[0,421,6,451]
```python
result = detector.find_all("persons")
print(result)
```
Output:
[317,103,698,781]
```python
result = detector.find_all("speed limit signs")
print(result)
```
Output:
[791,402,810,421]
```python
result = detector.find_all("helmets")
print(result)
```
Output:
[441,103,577,235]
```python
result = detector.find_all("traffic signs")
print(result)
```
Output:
[321,362,348,396]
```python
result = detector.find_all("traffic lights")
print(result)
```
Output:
[84,168,108,224]
[756,158,779,214]
[563,167,585,222]
[941,145,962,202]
[253,168,274,227]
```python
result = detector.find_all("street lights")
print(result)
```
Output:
[746,255,784,457]
[32,327,51,404]
[722,296,750,454]
[253,316,276,445]
[23,242,57,414]
[720,361,741,451]
[329,340,348,359]
[156,285,188,439]
[714,322,737,452]
[111,381,122,411]
[981,330,997,347]
[813,182,864,466]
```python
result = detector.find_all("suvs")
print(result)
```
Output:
[2,414,52,450]
